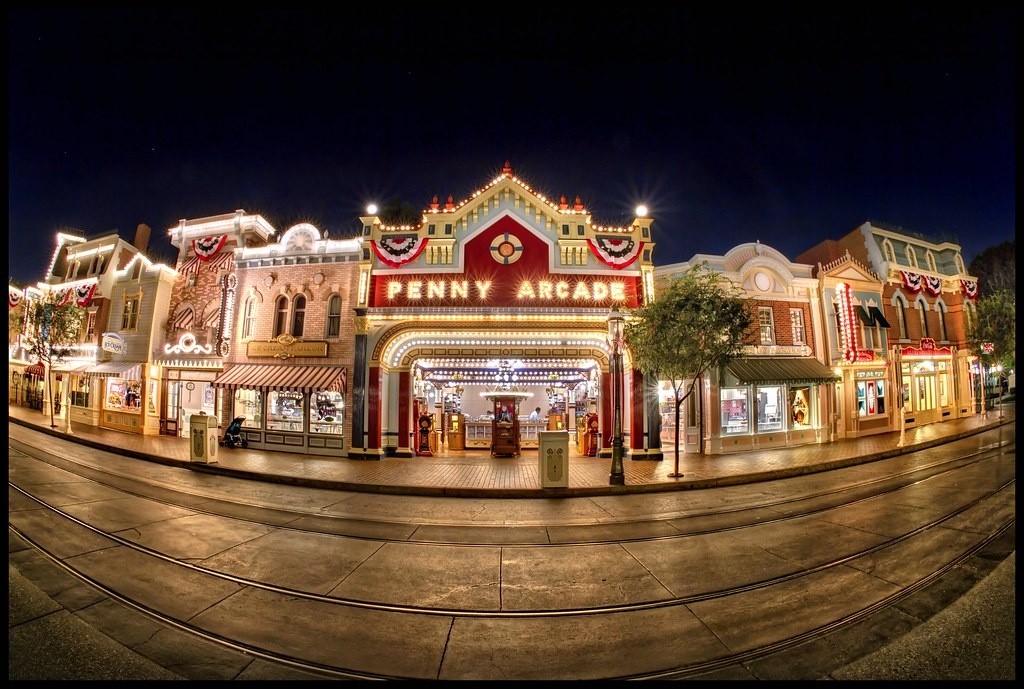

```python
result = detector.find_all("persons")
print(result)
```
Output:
[529,407,542,422]
[487,411,491,415]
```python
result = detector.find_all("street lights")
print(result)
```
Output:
[605,303,628,487]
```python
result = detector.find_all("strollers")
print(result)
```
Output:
[218,415,249,449]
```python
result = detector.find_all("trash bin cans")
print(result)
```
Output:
[537,429,569,488]
[189,411,219,465]
